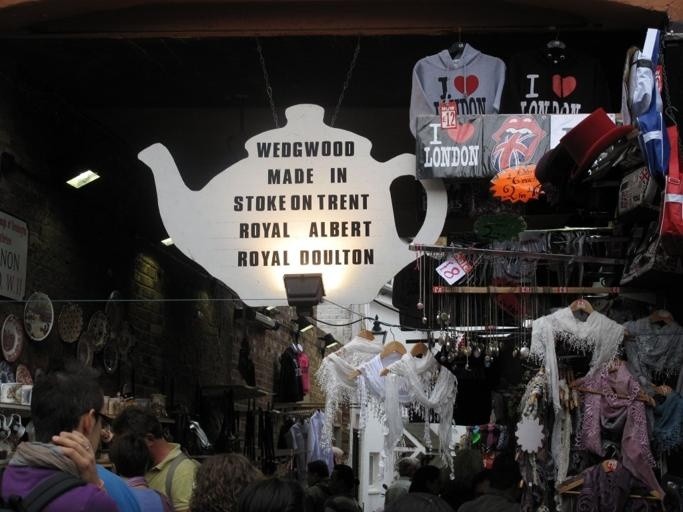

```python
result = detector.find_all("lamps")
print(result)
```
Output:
[290,317,343,359]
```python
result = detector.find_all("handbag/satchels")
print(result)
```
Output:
[622,47,663,127]
[636,109,669,177]
[620,228,683,284]
[473,212,527,242]
[619,167,657,217]
[658,174,683,237]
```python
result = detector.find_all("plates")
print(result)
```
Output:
[0,360,33,385]
[57,302,85,343]
[1,313,25,363]
[23,291,55,343]
[87,290,138,374]
[77,331,94,369]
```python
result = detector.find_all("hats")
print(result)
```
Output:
[560,108,634,182]
[536,147,590,206]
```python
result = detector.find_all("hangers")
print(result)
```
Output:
[550,446,670,501]
[325,318,459,381]
[530,295,682,412]
